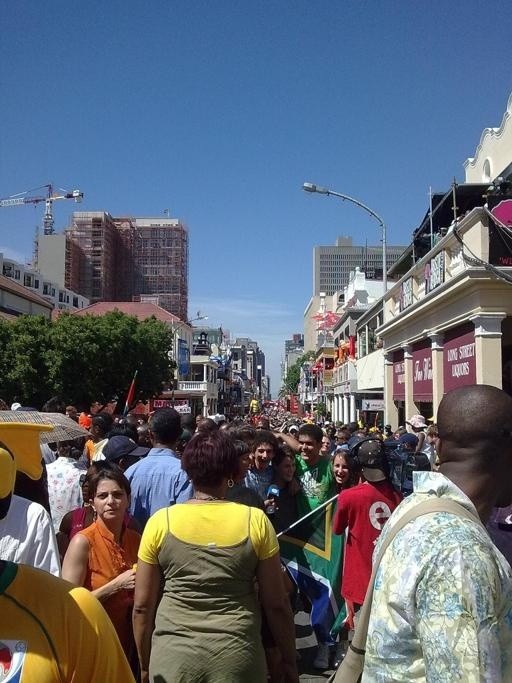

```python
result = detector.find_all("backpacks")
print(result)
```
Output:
[326,498,491,683]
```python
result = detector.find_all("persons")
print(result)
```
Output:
[1,392,404,436]
[59,468,142,674]
[0,440,61,578]
[43,429,89,533]
[332,437,402,630]
[123,406,195,523]
[242,430,278,501]
[360,384,512,681]
[226,438,280,515]
[398,432,419,454]
[132,432,299,683]
[102,434,150,471]
[406,414,435,468]
[272,446,295,491]
[318,434,334,457]
[0,558,137,683]
[293,422,335,534]
[311,449,358,667]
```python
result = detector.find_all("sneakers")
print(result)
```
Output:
[335,638,350,662]
[313,642,332,668]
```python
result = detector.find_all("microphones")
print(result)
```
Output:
[265,483,280,516]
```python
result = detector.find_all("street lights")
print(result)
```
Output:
[173,315,208,408]
[304,183,388,294]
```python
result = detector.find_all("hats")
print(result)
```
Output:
[356,439,389,483]
[325,421,330,425]
[407,414,428,428]
[100,433,151,460]
[289,425,299,432]
[348,436,367,451]
[395,433,419,445]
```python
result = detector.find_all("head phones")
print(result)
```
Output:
[347,437,385,464]
